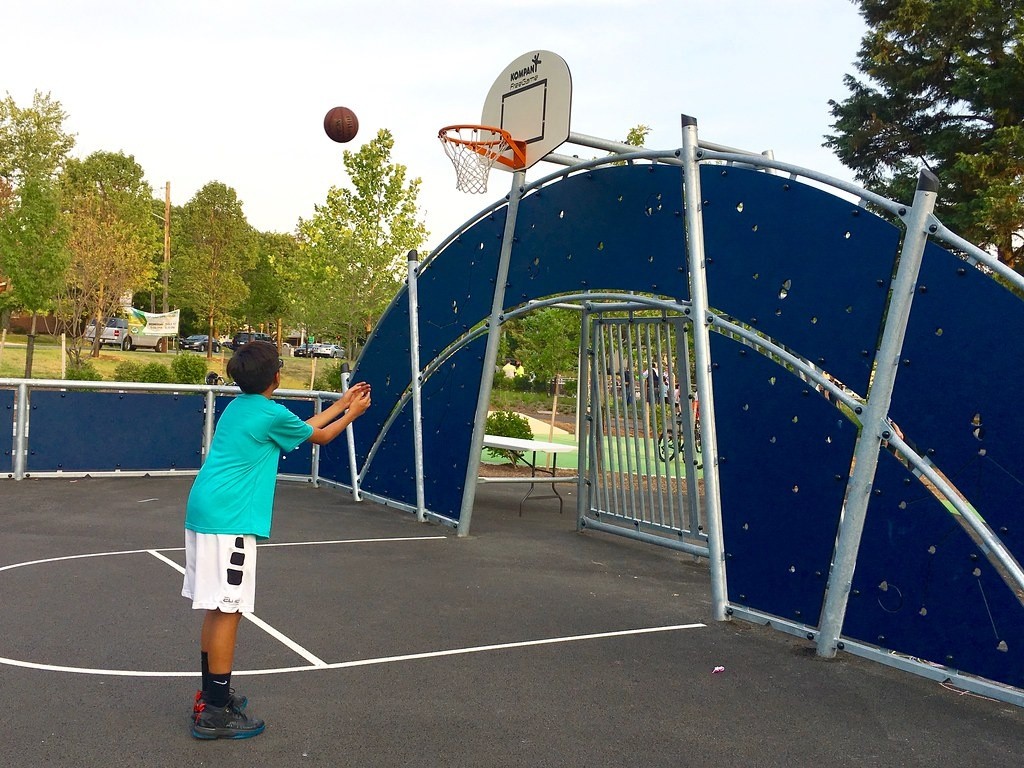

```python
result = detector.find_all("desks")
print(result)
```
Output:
[483,434,578,517]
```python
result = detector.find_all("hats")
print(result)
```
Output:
[506,358,511,362]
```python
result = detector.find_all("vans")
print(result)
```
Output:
[232,333,278,352]
[83,318,163,353]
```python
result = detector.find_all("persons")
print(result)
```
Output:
[596,361,699,416]
[492,357,525,381]
[180,341,370,741]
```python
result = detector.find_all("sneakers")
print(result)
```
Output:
[191,688,265,740]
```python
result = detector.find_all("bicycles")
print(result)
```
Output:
[659,418,705,470]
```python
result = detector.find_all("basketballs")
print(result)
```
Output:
[323,106,359,144]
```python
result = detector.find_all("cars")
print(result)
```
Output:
[179,335,220,353]
[313,344,346,359]
[294,343,320,359]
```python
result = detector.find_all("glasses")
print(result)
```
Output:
[276,357,284,368]
